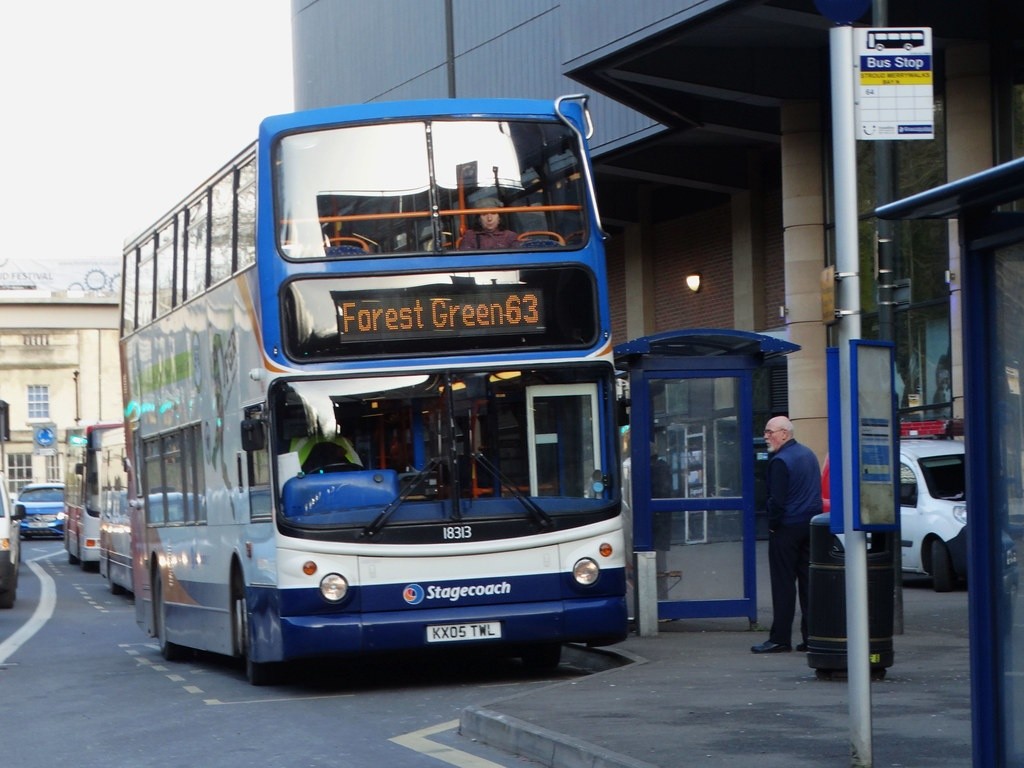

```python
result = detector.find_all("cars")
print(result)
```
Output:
[816,437,968,593]
[16,482,66,539]
[0,469,26,608]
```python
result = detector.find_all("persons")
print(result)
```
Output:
[650,443,678,623]
[751,416,823,653]
[460,198,524,251]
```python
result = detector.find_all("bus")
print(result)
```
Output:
[115,103,633,682]
[63,423,135,599]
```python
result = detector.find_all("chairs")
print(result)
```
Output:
[288,436,365,474]
[514,231,566,247]
[322,237,371,255]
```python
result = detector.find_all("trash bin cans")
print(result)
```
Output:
[806,511,895,683]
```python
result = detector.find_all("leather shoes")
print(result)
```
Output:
[796,643,808,652]
[751,641,792,653]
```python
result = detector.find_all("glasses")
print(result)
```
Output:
[764,429,783,436]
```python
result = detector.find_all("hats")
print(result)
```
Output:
[474,197,504,208]
